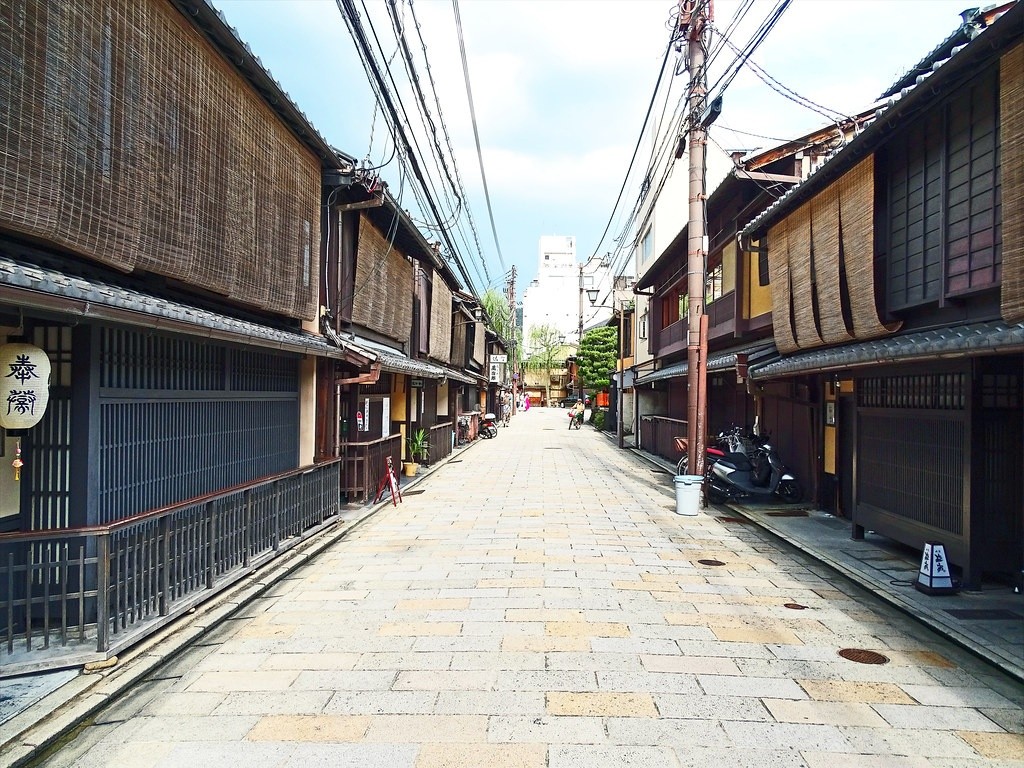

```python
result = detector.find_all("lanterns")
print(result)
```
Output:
[0,343,52,438]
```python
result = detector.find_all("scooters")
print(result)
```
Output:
[708,429,803,505]
[674,426,760,486]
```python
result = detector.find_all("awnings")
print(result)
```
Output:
[0,258,480,388]
[633,137,802,297]
[739,0,1024,251]
[339,180,463,291]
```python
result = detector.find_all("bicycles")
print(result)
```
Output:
[567,409,583,430]
[478,416,498,439]
[453,422,466,445]
[503,411,510,428]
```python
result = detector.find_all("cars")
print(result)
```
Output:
[560,392,591,408]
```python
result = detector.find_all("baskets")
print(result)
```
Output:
[569,413,575,417]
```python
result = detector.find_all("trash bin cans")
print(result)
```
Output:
[672,474,705,516]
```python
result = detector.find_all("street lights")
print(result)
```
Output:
[559,335,583,402]
[587,287,624,449]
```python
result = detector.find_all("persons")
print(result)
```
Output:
[516,392,520,408]
[571,399,585,425]
[524,393,530,411]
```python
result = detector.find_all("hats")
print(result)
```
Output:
[577,399,582,402]
